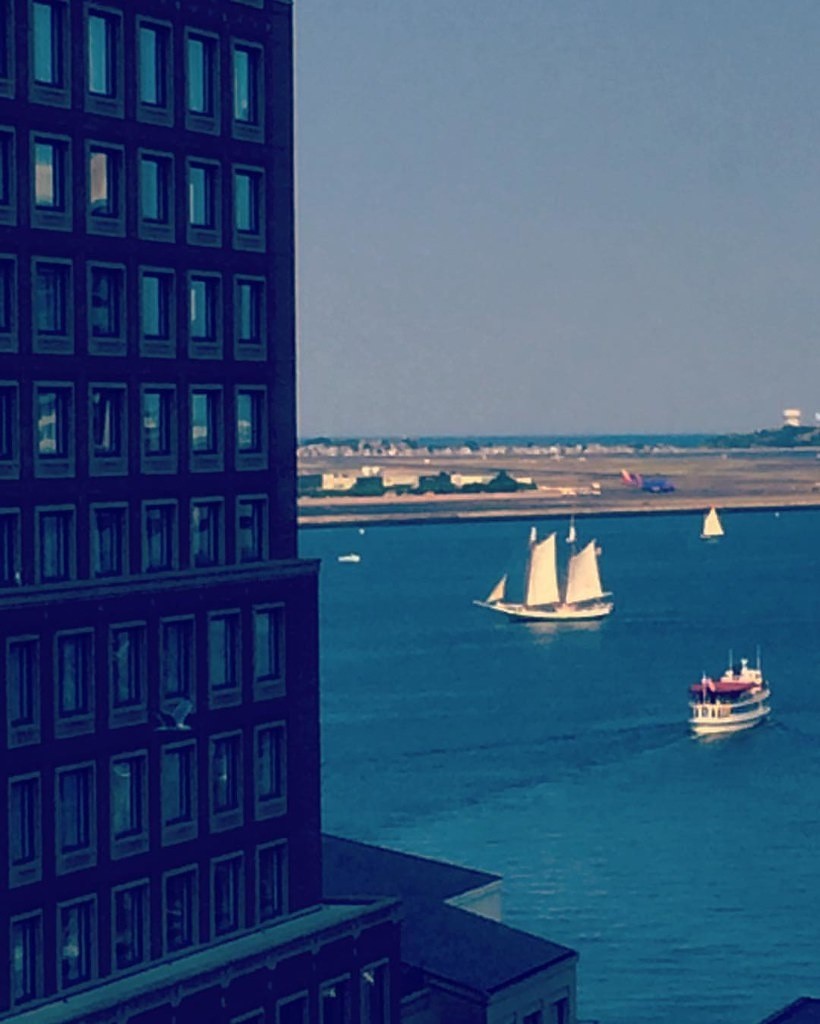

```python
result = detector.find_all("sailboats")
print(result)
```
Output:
[700,507,725,539]
[472,531,615,620]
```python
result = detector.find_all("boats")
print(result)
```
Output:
[687,645,772,742]
